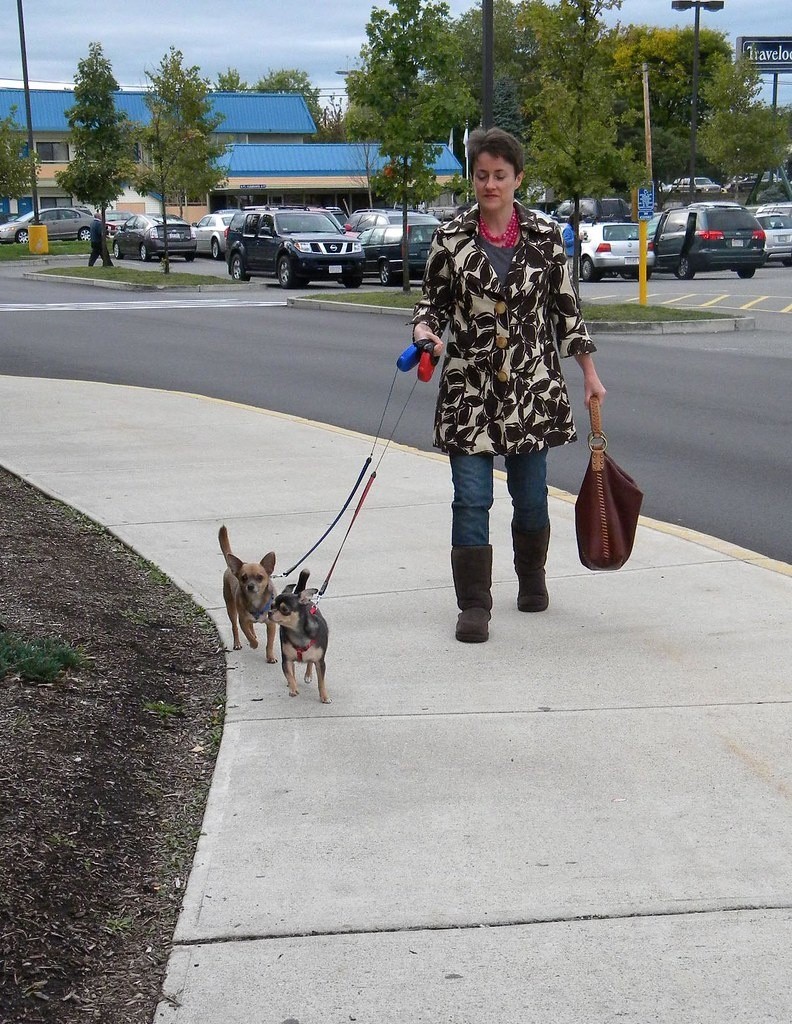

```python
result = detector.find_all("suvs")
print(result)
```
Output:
[340,209,441,233]
[672,176,721,193]
[224,204,366,290]
[754,201,792,222]
[631,201,770,280]
[552,196,633,224]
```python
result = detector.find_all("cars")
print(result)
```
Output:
[354,222,441,287]
[93,209,135,238]
[112,212,199,263]
[189,212,235,261]
[0,206,94,245]
[212,203,471,224]
[751,211,792,268]
[554,219,656,283]
[720,170,783,194]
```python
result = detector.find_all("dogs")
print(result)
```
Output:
[267,569,332,705]
[218,525,279,664]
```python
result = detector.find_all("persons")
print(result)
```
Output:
[88,213,114,266]
[562,213,585,302]
[406,127,606,643]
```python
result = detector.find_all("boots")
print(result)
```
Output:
[512,524,553,612]
[448,543,497,642]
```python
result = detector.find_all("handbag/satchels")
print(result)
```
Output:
[575,396,644,570]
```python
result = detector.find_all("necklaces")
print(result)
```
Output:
[478,210,519,248]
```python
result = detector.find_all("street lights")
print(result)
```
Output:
[671,0,725,204]
